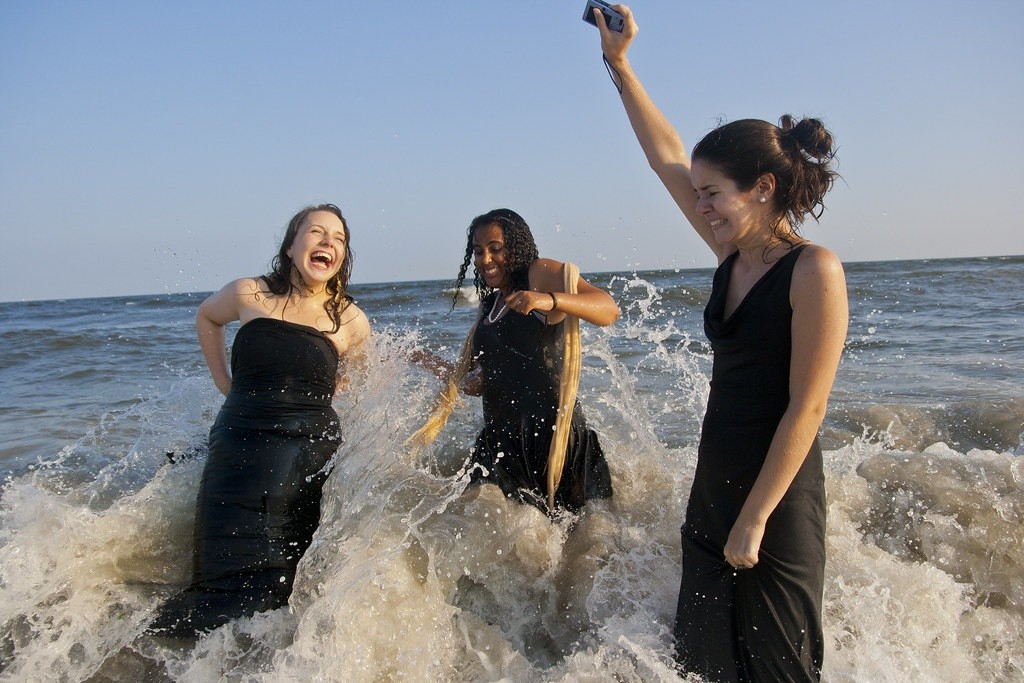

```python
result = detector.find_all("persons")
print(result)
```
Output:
[406,207,620,516]
[594,2,850,683]
[183,200,370,623]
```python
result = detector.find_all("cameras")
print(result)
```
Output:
[582,0,624,33]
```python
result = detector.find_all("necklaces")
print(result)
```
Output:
[487,288,516,323]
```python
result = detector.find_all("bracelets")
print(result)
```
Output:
[545,289,556,314]
